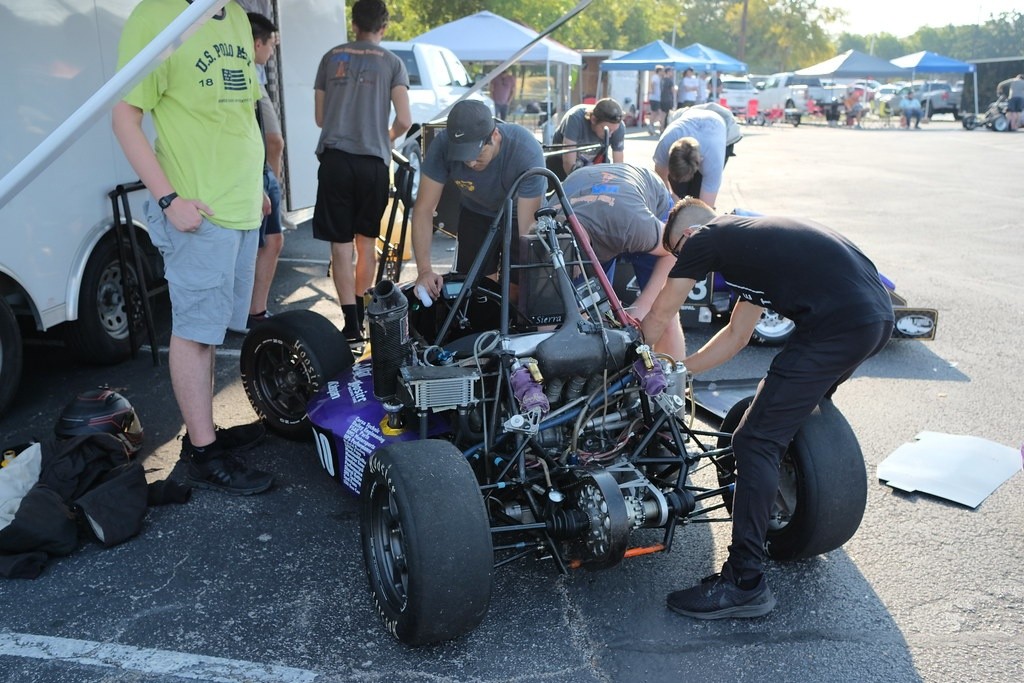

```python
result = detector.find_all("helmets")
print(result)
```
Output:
[54,389,146,462]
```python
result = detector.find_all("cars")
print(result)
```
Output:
[824,78,926,102]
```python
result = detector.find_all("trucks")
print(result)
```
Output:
[0,0,350,412]
[557,48,652,128]
[959,57,1024,128]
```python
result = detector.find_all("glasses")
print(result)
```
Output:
[671,235,685,258]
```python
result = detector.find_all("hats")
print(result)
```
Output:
[445,99,495,161]
[595,98,622,123]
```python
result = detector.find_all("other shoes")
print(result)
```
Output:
[245,311,270,328]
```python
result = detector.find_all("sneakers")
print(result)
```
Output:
[184,448,274,495]
[668,561,777,619]
[181,420,265,462]
[340,323,366,357]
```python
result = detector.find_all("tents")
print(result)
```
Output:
[672,42,750,128]
[792,49,980,119]
[407,10,582,147]
[594,39,718,129]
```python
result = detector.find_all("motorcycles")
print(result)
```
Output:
[964,93,1011,132]
[240,168,871,647]
[577,203,939,349]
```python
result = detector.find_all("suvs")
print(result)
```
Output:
[377,39,499,208]
[717,74,759,117]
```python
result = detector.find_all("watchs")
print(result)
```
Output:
[159,192,178,208]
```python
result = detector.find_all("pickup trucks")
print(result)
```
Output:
[887,81,951,118]
[946,79,965,121]
[758,73,850,128]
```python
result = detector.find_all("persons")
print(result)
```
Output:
[898,90,923,130]
[844,92,862,129]
[640,196,896,621]
[489,69,517,121]
[647,64,724,136]
[995,73,1024,131]
[311,0,414,350]
[411,98,742,479]
[111,0,286,498]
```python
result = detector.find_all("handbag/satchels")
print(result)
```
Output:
[10,431,150,553]
